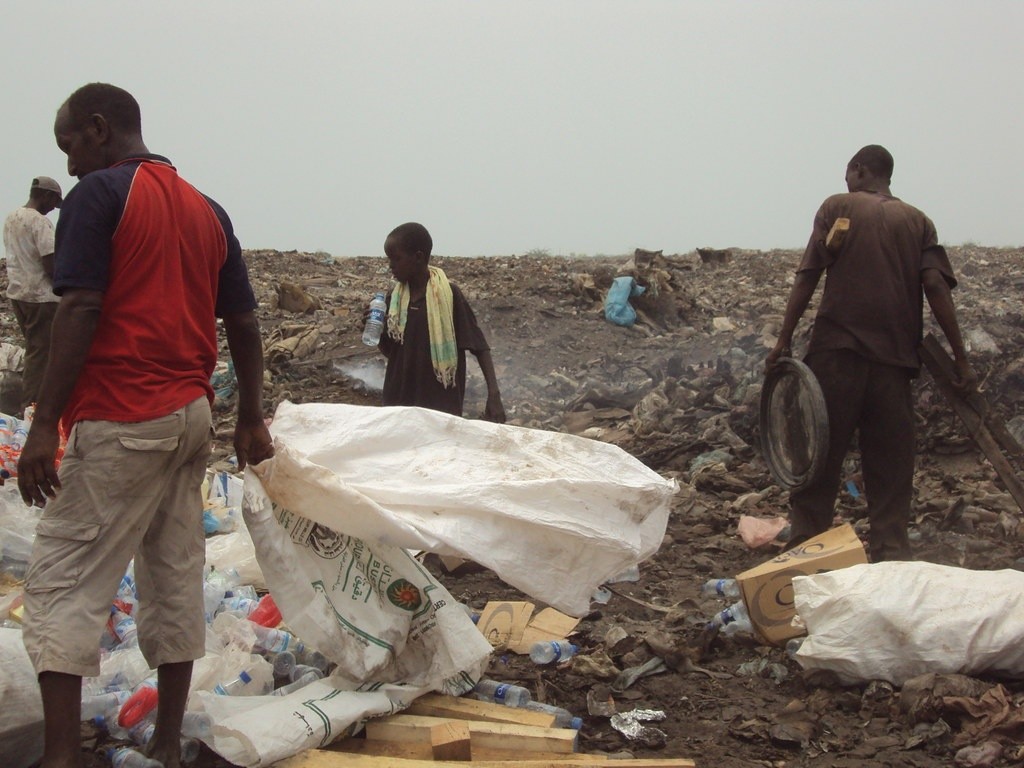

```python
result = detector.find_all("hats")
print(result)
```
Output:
[31,176,64,209]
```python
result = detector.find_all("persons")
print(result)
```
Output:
[359,222,508,427]
[764,143,976,577]
[3,175,69,417]
[14,80,279,767]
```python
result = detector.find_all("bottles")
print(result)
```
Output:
[706,600,747,630]
[706,578,743,600]
[0,413,591,734]
[362,292,387,347]
